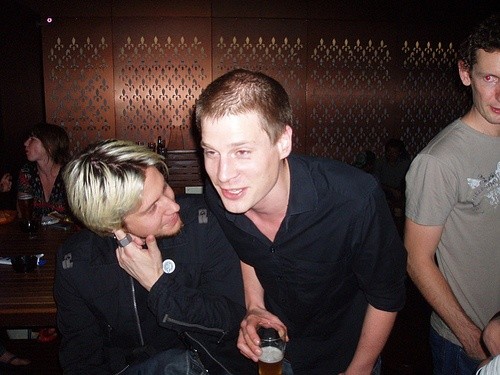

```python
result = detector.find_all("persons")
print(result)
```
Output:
[52,136,294,375]
[0,123,75,226]
[195,70,408,375]
[404,21,500,375]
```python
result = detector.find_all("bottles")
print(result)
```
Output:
[161,140,167,156]
[157,137,162,155]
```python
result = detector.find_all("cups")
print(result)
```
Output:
[258,323,286,375]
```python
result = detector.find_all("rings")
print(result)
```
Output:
[116,234,132,247]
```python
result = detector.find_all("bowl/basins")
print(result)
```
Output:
[11,255,37,272]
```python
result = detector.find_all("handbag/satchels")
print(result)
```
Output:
[113,346,209,375]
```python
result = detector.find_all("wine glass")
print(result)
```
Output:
[17,194,40,240]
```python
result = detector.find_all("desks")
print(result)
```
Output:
[0,216,89,329]
